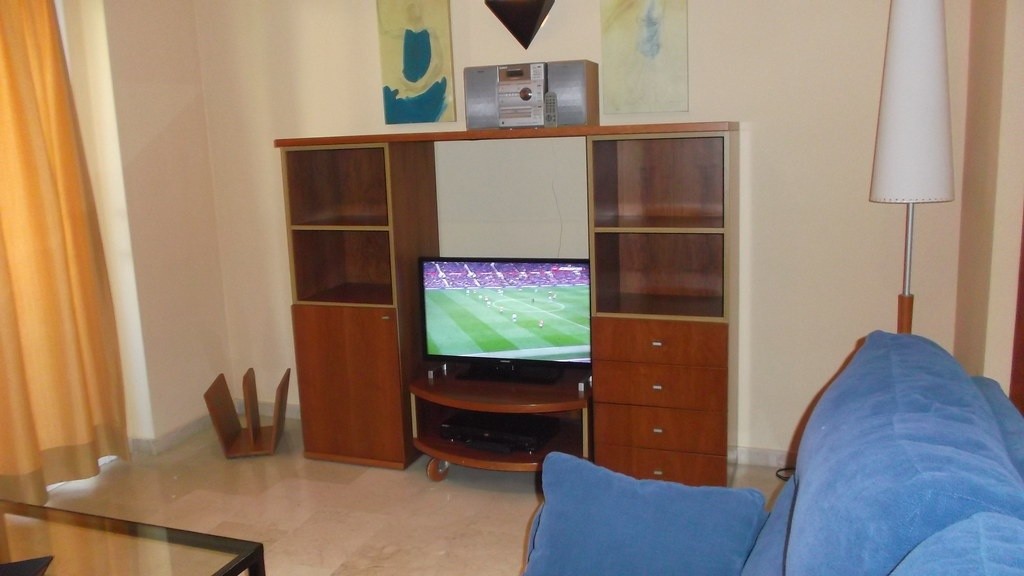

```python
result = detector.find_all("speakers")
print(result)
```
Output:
[464,66,500,131]
[548,59,599,126]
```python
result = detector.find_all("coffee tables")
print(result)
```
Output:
[0,498,266,576]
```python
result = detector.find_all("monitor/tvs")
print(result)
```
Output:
[417,256,592,385]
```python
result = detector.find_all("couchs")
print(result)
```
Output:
[526,329,1024,576]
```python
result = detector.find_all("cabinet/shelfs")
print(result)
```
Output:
[271,119,742,489]
[407,367,592,484]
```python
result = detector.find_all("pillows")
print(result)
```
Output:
[523,452,766,576]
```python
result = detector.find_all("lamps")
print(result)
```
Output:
[869,0,956,334]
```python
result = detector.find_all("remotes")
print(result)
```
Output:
[544,92,558,128]
